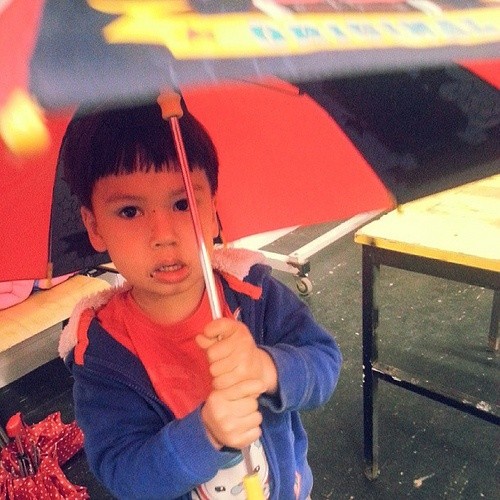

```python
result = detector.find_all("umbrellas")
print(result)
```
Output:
[0,0,500,482]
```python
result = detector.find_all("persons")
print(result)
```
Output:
[55,97,343,500]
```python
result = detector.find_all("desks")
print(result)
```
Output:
[352,169,500,484]
